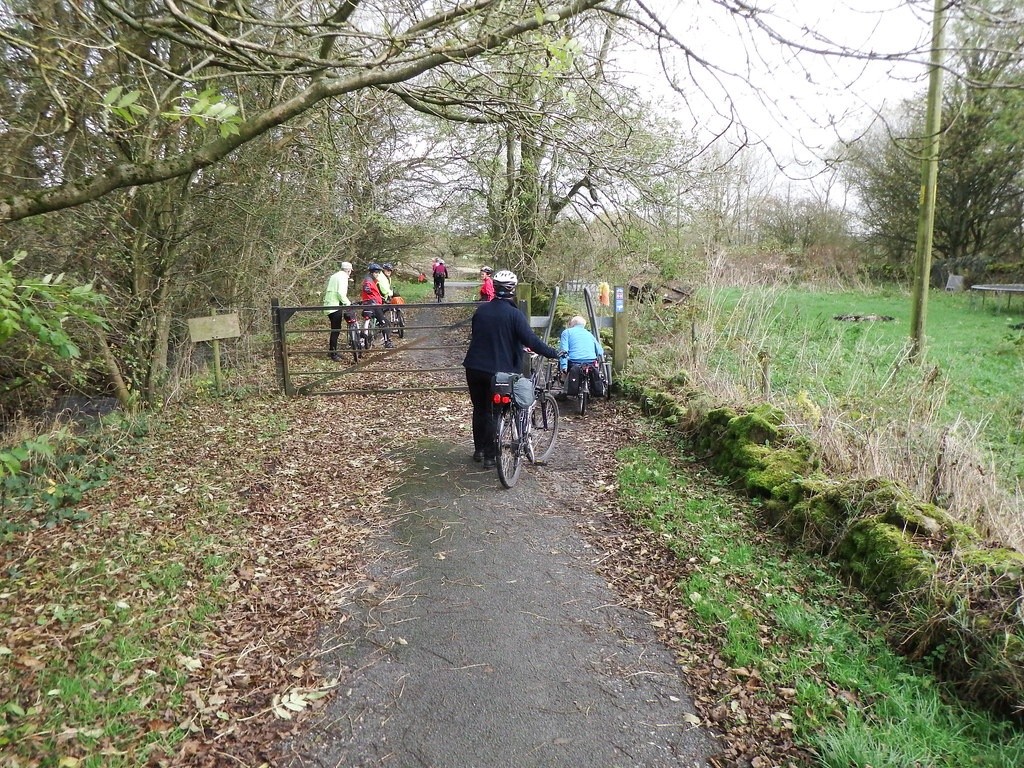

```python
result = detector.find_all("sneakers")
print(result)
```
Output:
[483,457,496,468]
[473,451,485,462]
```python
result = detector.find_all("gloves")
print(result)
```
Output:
[555,348,566,359]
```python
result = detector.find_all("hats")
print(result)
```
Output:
[341,262,353,271]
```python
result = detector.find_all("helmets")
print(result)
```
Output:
[435,257,439,261]
[381,263,392,271]
[369,264,383,270]
[481,266,493,276]
[439,260,445,264]
[493,270,518,291]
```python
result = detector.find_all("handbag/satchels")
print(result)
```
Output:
[390,297,404,304]
[490,371,536,409]
[589,365,605,397]
[562,364,582,396]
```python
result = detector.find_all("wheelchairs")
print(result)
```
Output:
[547,355,612,415]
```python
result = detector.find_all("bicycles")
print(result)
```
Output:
[433,276,443,302]
[383,292,406,338]
[341,299,374,361]
[491,351,568,489]
[362,299,379,349]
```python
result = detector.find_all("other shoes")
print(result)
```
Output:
[385,342,397,348]
[331,355,342,362]
[328,352,343,359]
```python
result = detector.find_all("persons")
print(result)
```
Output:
[461,270,566,469]
[434,262,448,298]
[324,262,354,362]
[433,258,440,271]
[377,263,400,304]
[558,316,604,383]
[361,264,397,348]
[480,266,496,301]
